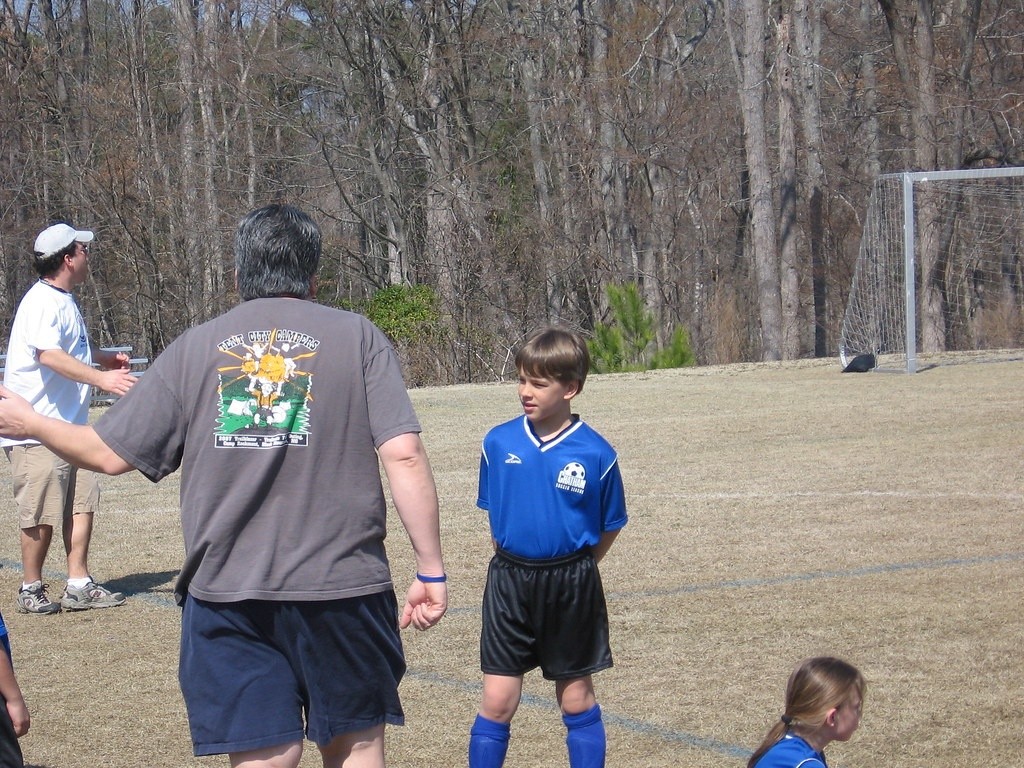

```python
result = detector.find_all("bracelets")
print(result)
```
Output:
[416,573,447,583]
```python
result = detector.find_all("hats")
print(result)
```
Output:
[33,224,94,260]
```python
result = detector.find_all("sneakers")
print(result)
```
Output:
[61,582,126,609]
[16,583,61,614]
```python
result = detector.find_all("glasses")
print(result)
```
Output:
[66,245,88,257]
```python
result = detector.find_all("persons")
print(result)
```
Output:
[0,614,30,768]
[467,327,628,768]
[746,655,866,768]
[0,223,138,614]
[0,204,448,768]
